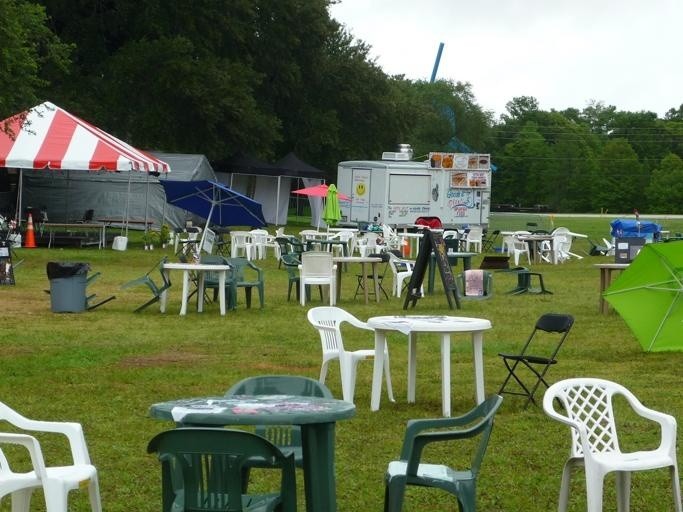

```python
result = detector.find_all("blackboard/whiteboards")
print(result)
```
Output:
[431,237,458,291]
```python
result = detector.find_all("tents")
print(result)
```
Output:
[21,152,218,233]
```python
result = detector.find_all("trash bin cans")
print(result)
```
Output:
[47,263,88,313]
[444,239,458,266]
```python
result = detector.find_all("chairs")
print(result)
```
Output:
[0,397,106,512]
[4,229,26,271]
[121,255,171,312]
[160,216,499,316]
[86,271,116,310]
[380,387,504,510]
[501,223,588,265]
[208,369,335,494]
[498,314,577,412]
[495,267,554,296]
[143,422,302,511]
[590,238,615,256]
[304,301,400,407]
[44,289,52,295]
[536,376,682,512]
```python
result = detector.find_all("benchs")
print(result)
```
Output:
[95,220,153,233]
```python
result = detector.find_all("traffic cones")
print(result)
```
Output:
[23,214,39,248]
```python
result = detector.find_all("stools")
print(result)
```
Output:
[660,231,669,241]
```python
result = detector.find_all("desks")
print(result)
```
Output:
[364,311,492,413]
[146,396,358,512]
[595,264,631,314]
[46,223,108,249]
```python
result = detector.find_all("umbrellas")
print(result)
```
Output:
[601,240,683,354]
[291,184,351,232]
[322,184,342,253]
[158,180,268,258]
